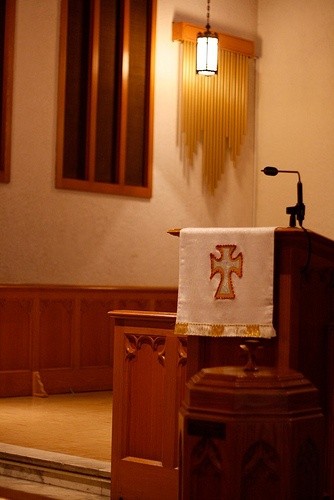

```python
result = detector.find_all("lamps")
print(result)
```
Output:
[194,0,220,78]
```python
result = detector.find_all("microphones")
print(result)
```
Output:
[262,167,306,228]
